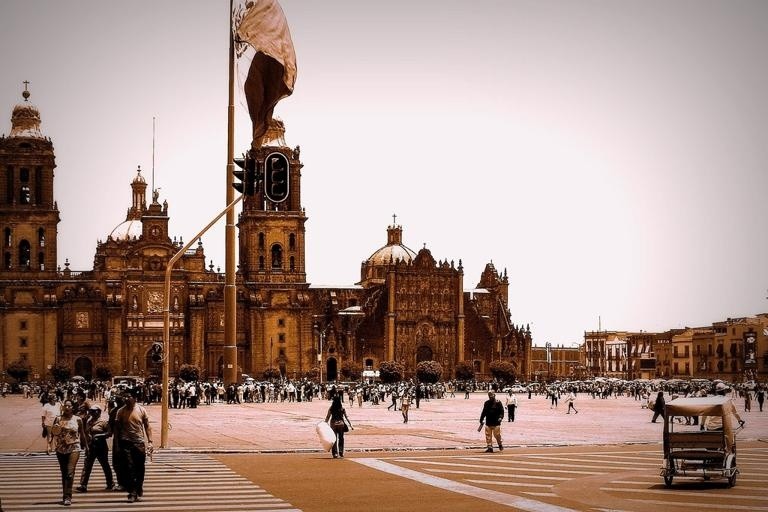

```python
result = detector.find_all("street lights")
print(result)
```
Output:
[314,324,331,384]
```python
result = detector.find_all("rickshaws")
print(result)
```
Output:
[659,397,745,487]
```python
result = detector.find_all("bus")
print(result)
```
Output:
[565,381,593,390]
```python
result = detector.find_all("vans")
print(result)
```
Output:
[340,382,358,391]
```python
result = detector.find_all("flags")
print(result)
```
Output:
[231,1,298,151]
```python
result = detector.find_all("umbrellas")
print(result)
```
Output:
[71,375,85,382]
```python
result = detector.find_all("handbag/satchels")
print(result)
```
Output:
[42,427,45,438]
[335,420,345,428]
[51,423,62,437]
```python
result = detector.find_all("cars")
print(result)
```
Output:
[502,385,527,393]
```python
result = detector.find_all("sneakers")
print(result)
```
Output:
[76,485,87,493]
[114,485,125,492]
[499,444,504,451]
[485,448,492,453]
[63,498,71,505]
[339,451,344,457]
[333,454,338,458]
[127,493,144,503]
[106,481,115,489]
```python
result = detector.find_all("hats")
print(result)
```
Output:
[89,405,102,412]
[716,383,729,391]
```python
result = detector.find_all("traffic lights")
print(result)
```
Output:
[151,342,163,364]
[262,151,290,203]
[232,149,259,202]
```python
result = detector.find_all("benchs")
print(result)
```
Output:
[669,430,725,470]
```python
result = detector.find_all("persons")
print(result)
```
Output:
[19,379,162,505]
[700,382,744,450]
[400,389,411,424]
[505,389,518,422]
[325,396,354,459]
[168,377,471,411]
[479,389,505,453]
[526,376,768,423]
